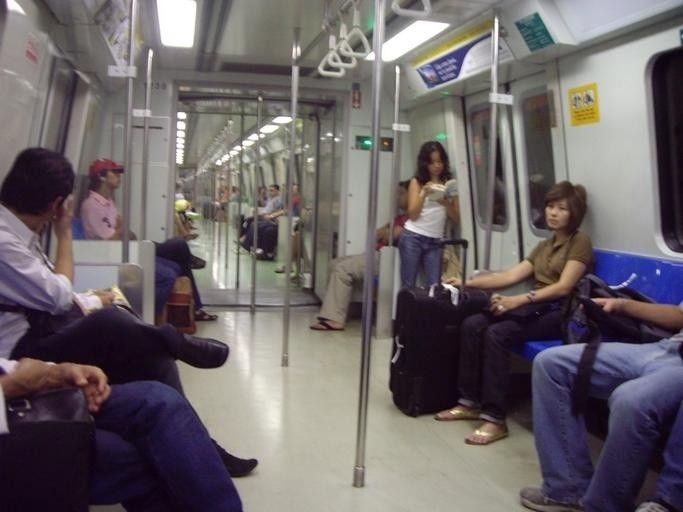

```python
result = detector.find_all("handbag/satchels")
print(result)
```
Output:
[3,385,98,512]
[0,304,50,337]
[559,273,674,347]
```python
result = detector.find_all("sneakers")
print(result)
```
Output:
[274,265,293,273]
[519,486,583,511]
[184,225,199,241]
[233,239,273,259]
[634,500,669,512]
[189,256,205,269]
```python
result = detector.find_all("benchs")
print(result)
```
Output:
[512,245,682,362]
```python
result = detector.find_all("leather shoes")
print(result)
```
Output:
[222,452,257,476]
[172,332,228,368]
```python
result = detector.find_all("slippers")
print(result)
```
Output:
[435,405,480,420]
[309,320,343,330]
[464,425,508,444]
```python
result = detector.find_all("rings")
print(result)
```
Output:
[495,305,504,310]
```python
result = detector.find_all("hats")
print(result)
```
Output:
[87,158,124,175]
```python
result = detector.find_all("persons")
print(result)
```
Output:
[203,180,302,260]
[519,297,683,512]
[434,181,594,445]
[174,181,200,241]
[309,179,410,330]
[1,356,244,512]
[392,139,461,291]
[635,402,682,512]
[1,148,258,476]
[66,216,182,326]
[80,158,218,321]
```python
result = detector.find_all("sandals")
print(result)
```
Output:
[194,310,217,321]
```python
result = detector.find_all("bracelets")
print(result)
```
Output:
[524,291,538,305]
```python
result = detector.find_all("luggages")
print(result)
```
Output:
[388,239,490,416]
